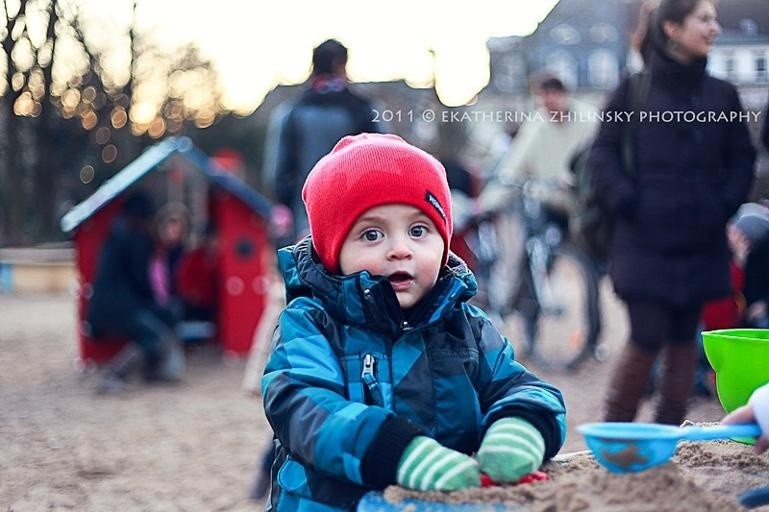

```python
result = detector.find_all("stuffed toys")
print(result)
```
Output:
[478,469,552,487]
[575,421,762,477]
[697,327,769,447]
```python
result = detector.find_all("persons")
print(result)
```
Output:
[430,74,614,370]
[261,35,381,308]
[719,381,769,455]
[257,129,570,510]
[80,199,198,394]
[642,101,768,400]
[567,1,761,419]
[82,191,162,341]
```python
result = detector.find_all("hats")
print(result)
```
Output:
[302,130,454,276]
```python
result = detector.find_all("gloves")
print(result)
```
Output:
[396,434,482,492]
[476,414,546,484]
